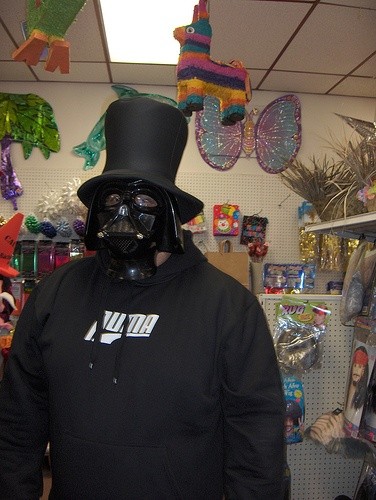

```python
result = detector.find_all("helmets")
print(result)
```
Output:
[84,178,184,282]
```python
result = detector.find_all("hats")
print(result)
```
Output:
[77,97,204,226]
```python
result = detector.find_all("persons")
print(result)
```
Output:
[1,94,288,496]
